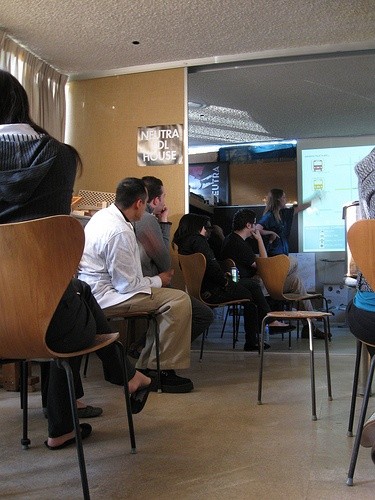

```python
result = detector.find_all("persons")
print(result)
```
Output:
[255,189,326,259]
[129,176,217,363]
[0,67,158,452]
[172,213,295,351]
[217,208,334,340]
[39,359,103,420]
[199,215,226,254]
[69,177,195,394]
[347,146,375,464]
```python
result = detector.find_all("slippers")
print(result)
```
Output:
[45,423,92,449]
[130,371,155,414]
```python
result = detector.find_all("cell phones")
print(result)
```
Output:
[230,267,238,283]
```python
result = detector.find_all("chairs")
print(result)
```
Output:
[0,215,137,500]
[256,254,332,351]
[344,219,375,486]
[80,311,160,379]
[176,253,251,363]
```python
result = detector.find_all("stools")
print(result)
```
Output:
[256,310,333,421]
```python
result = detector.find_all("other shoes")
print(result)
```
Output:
[77,405,103,418]
[244,342,272,352]
[301,326,332,339]
[269,324,296,335]
[146,369,194,394]
[360,412,375,448]
